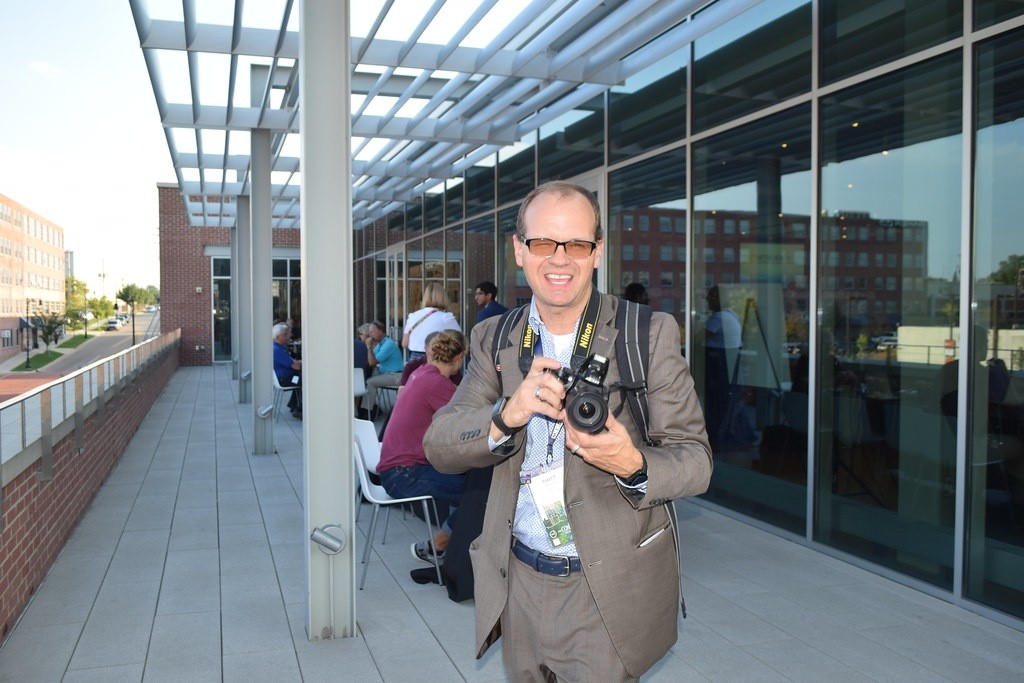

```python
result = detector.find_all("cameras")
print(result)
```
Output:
[555,353,611,436]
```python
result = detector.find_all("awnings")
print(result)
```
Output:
[21,317,35,328]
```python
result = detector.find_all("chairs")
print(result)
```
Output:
[354,435,446,587]
[375,385,399,420]
[354,418,415,524]
[354,367,371,420]
[272,367,302,422]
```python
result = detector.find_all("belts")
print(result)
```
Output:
[510,535,582,577]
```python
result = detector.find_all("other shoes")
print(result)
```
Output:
[357,405,382,422]
[292,409,301,418]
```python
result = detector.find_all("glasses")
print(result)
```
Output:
[520,235,597,259]
[474,292,484,297]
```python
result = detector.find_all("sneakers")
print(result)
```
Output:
[411,539,447,567]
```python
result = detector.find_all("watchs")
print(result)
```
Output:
[617,452,648,486]
[492,396,527,434]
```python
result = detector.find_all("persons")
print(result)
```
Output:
[626,279,957,553]
[274,320,302,418]
[354,323,405,420]
[402,283,462,360]
[423,182,713,683]
[475,283,509,321]
[54,332,59,345]
[376,329,467,566]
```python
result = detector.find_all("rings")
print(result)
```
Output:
[535,387,543,399]
[571,444,579,453]
